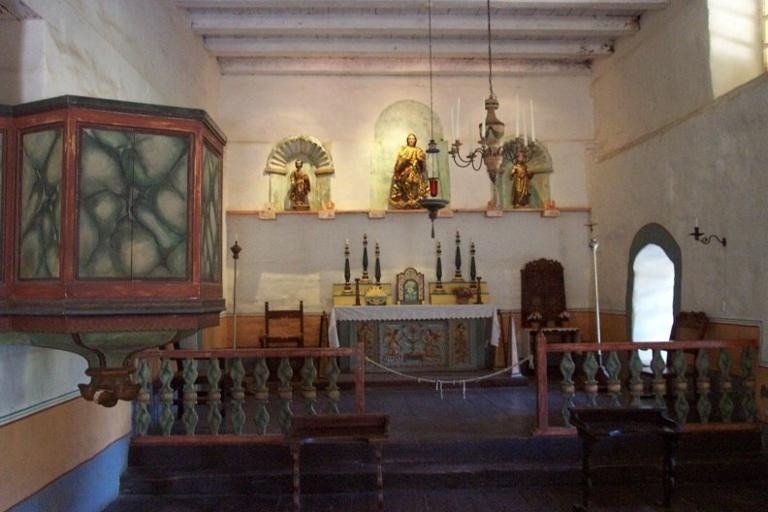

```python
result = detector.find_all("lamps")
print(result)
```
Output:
[422,0,449,238]
[448,0,538,184]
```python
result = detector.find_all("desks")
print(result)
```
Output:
[562,391,680,512]
[284,412,391,512]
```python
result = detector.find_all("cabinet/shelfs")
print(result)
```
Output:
[520,258,581,369]
[260,300,304,378]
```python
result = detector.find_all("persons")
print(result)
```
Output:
[288,159,312,210]
[423,329,440,356]
[389,132,429,209]
[387,329,402,355]
[510,151,534,209]
